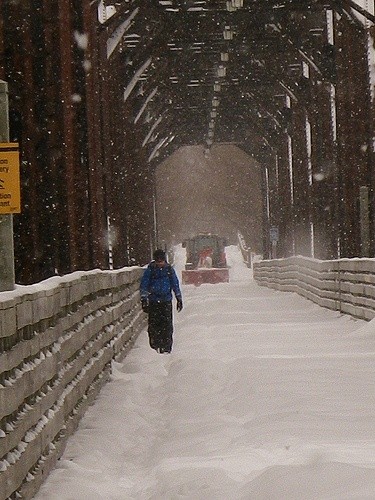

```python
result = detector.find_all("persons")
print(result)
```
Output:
[139,249,184,355]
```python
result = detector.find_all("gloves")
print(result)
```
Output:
[142,300,149,313]
[177,297,183,313]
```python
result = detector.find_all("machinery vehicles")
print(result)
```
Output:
[181,232,230,288]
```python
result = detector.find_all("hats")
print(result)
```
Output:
[153,248,165,262]
